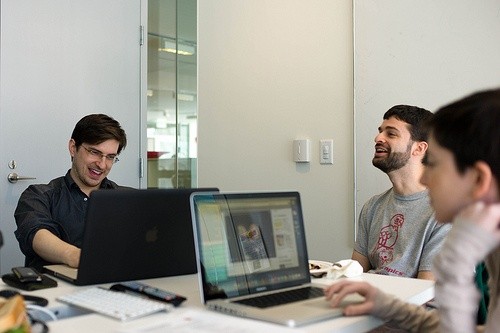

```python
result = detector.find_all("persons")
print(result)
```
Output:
[13,114,127,270]
[349,104,455,279]
[324,85,500,333]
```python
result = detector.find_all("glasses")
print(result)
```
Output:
[80,144,119,163]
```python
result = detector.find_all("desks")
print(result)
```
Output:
[0,269,435,333]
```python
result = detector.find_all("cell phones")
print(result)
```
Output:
[12,267,44,283]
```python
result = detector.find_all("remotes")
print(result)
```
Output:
[119,282,183,304]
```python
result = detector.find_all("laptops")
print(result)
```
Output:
[40,188,219,286]
[189,190,368,329]
